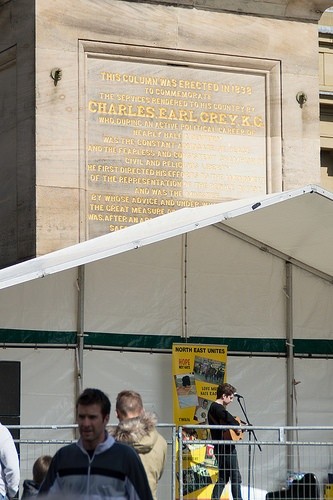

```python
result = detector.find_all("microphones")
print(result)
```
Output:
[234,392,243,398]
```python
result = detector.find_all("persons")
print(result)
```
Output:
[0,423,20,500]
[107,390,167,500]
[180,359,224,494]
[21,456,52,500]
[36,388,154,500]
[208,382,244,500]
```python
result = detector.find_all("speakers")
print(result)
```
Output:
[0,361,21,416]
[0,416,20,467]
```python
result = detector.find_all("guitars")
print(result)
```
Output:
[222,414,244,445]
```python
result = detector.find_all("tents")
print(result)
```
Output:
[0,185,333,498]
